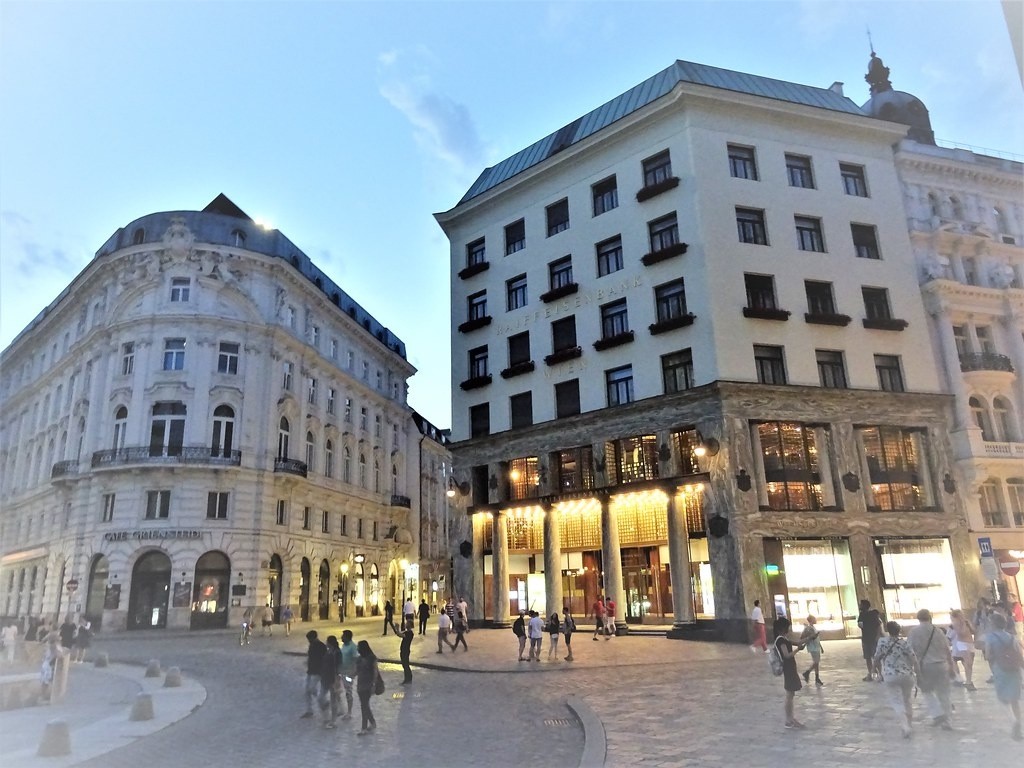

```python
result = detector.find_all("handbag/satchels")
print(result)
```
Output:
[552,633,559,639]
[426,612,430,618]
[800,626,815,639]
[374,672,385,695]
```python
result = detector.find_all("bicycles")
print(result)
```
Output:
[239,618,253,646]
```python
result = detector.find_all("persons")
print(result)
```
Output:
[0,614,93,705]
[261,603,274,638]
[383,598,471,684]
[512,595,616,663]
[300,629,378,735]
[749,599,1023,743]
[281,606,296,637]
[242,609,252,640]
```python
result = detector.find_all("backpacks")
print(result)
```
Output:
[513,620,523,636]
[460,619,467,630]
[768,636,788,675]
[992,632,1021,671]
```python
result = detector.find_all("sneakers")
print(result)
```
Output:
[785,719,805,729]
[765,649,770,653]
[750,645,756,653]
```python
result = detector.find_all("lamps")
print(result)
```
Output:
[446,476,470,497]
[693,429,720,458]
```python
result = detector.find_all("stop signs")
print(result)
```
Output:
[66,580,78,591]
[1000,556,1020,575]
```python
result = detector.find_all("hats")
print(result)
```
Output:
[518,610,525,614]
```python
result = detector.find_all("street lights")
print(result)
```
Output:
[340,562,349,622]
[399,559,407,631]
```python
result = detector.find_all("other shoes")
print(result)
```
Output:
[815,680,823,685]
[565,657,573,661]
[342,715,352,719]
[863,677,872,681]
[368,724,376,730]
[803,672,809,682]
[357,729,367,735]
[901,715,1024,741]
[326,724,337,727]
[300,712,313,718]
[966,682,976,691]
